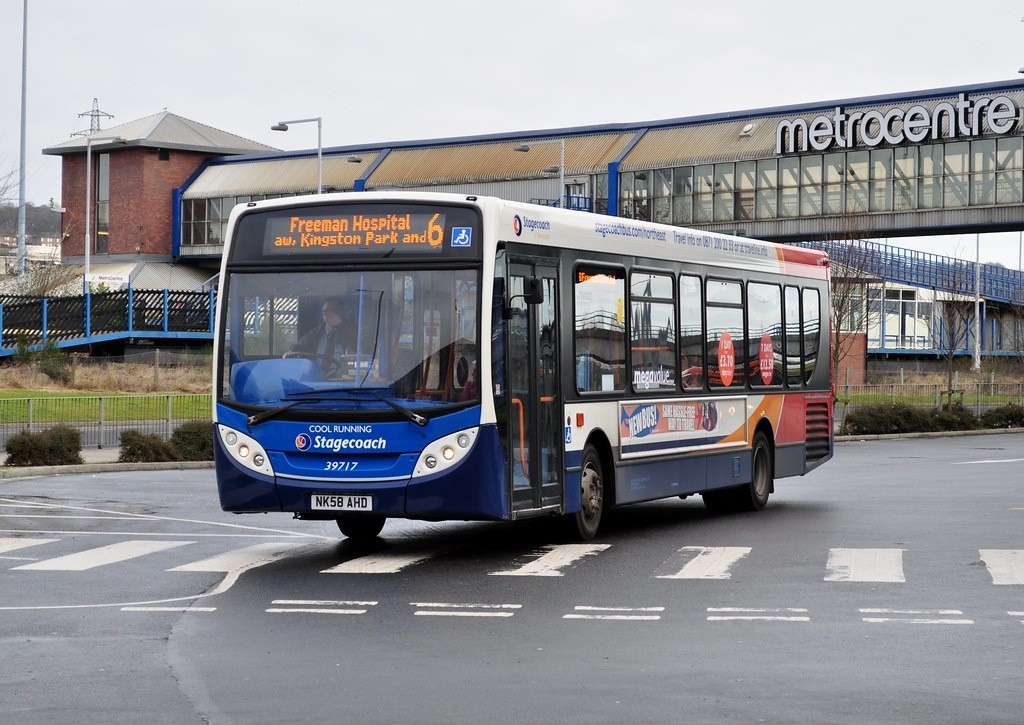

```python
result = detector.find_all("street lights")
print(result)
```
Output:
[84,137,127,292]
[512,138,565,209]
[271,116,362,195]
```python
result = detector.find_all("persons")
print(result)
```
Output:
[282,297,371,378]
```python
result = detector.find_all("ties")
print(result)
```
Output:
[322,332,334,374]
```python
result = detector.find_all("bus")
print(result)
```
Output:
[210,191,835,541]
